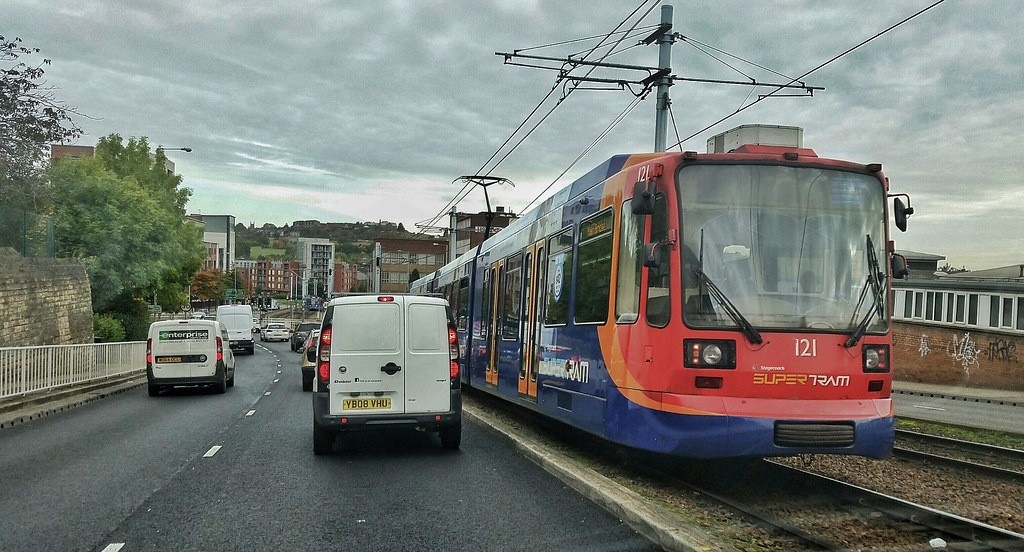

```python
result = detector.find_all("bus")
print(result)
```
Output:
[402,123,914,479]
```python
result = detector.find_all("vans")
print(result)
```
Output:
[145,319,237,396]
[215,303,256,355]
[306,290,464,456]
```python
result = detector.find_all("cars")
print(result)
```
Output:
[189,308,216,321]
[252,318,261,333]
[260,322,290,342]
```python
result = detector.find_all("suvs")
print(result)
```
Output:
[290,322,322,352]
[296,329,320,392]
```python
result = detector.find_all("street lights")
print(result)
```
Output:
[233,258,245,304]
